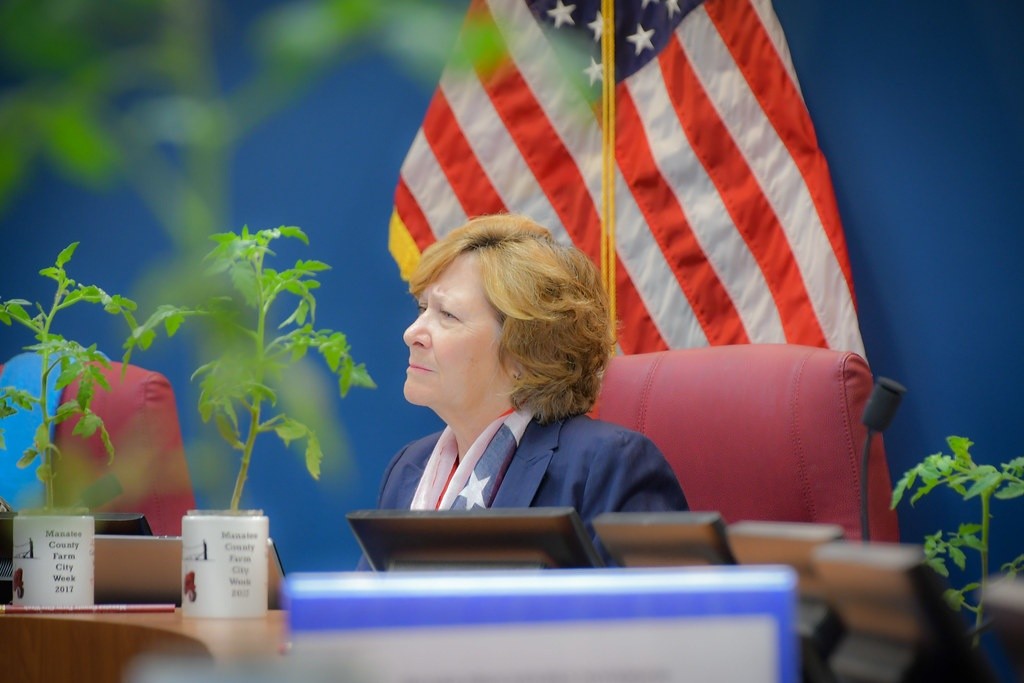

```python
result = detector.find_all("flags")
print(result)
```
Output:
[388,0,867,363]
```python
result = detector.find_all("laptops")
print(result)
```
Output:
[89,534,285,612]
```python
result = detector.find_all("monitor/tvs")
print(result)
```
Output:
[0,512,153,608]
[346,508,1024,683]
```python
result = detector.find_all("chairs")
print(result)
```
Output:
[0,347,191,526]
[586,347,895,542]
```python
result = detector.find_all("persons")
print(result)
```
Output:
[356,214,699,572]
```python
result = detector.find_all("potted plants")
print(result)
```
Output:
[0,241,138,610]
[121,225,373,614]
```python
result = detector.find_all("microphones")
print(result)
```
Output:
[860,376,905,540]
